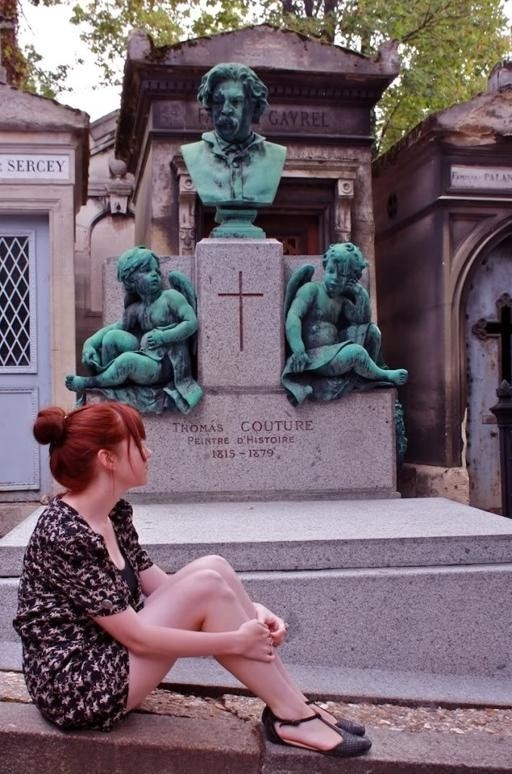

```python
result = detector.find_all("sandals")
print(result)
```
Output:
[262,700,372,758]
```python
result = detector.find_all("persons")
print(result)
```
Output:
[285,242,409,386]
[64,248,199,392]
[180,62,287,206]
[12,402,373,756]
